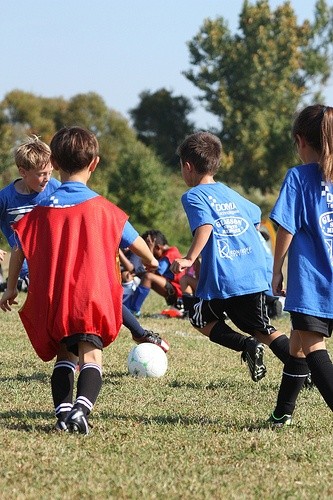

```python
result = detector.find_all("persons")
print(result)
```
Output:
[141,230,185,318]
[268,105,333,423]
[0,135,172,352]
[0,125,159,435]
[171,131,314,388]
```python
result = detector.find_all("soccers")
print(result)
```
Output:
[127,343,168,379]
